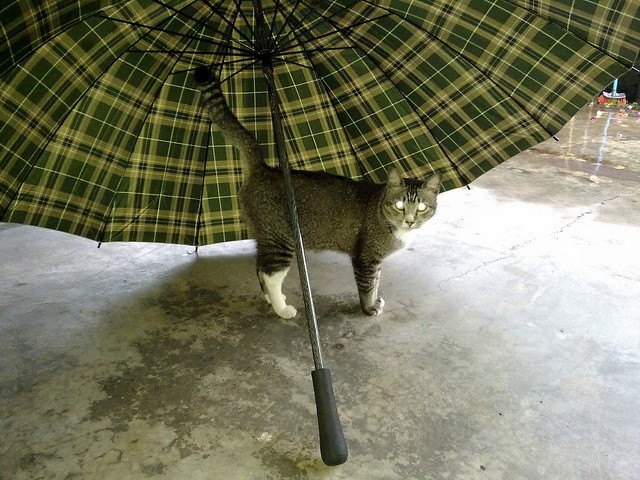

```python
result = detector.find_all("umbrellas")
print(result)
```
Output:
[0,0,640,465]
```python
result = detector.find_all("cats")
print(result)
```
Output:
[194,65,443,319]
